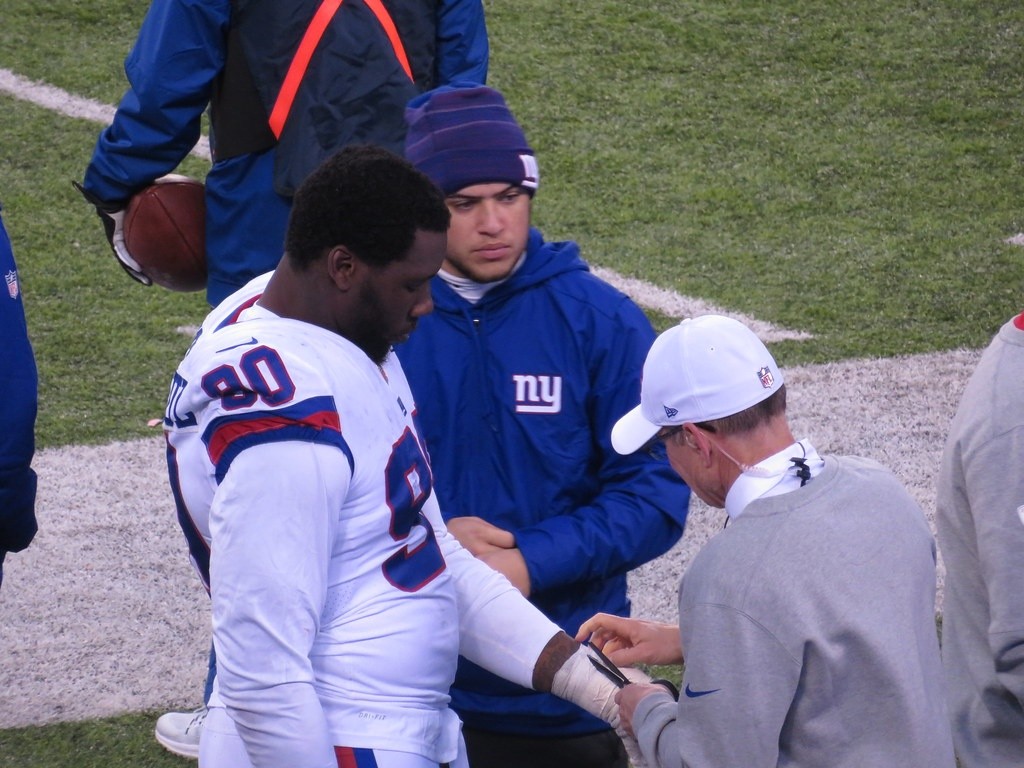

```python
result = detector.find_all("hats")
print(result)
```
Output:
[404,82,540,203]
[611,315,784,456]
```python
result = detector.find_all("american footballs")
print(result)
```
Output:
[123,174,208,293]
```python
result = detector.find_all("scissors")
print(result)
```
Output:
[586,638,679,702]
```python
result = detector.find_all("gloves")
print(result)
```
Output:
[71,180,158,287]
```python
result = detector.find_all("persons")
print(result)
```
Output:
[392,81,692,768]
[935,313,1024,768]
[578,315,954,768]
[167,144,671,768]
[0,212,38,572]
[70,0,490,758]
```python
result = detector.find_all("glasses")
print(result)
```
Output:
[642,422,717,463]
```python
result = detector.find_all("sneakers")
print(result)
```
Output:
[156,707,207,759]
